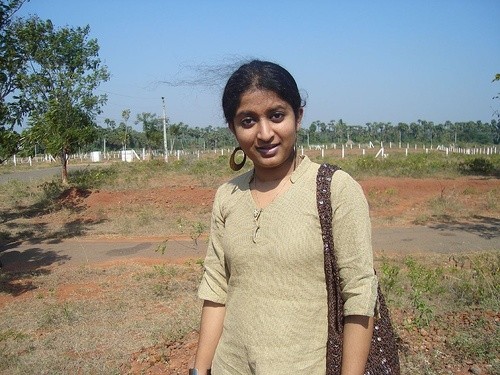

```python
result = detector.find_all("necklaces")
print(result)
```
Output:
[252,156,302,219]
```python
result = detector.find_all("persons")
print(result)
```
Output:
[189,60,378,375]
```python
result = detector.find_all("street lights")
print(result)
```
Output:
[161,96,169,162]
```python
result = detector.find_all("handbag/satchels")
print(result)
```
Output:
[316,164,401,375]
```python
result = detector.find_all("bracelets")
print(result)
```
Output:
[189,368,211,375]
